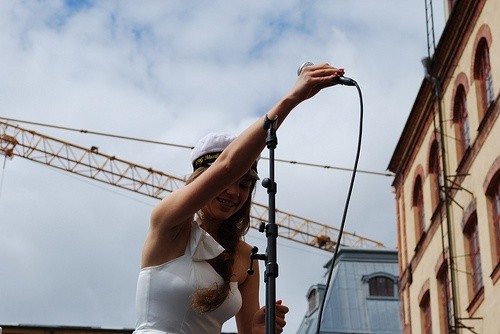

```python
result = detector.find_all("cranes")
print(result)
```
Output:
[0,117,395,254]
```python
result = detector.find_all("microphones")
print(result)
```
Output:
[297,62,356,86]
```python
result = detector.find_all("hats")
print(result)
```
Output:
[192,135,261,180]
